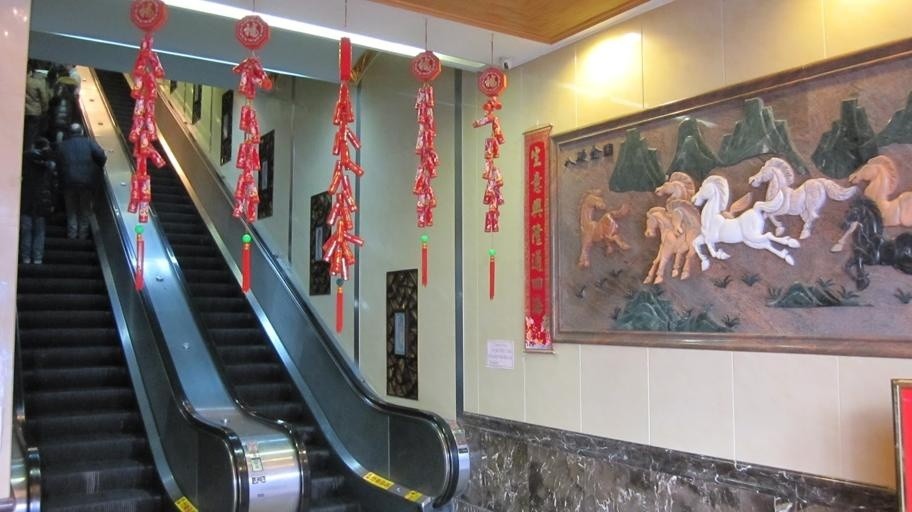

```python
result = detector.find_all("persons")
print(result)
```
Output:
[21,62,107,264]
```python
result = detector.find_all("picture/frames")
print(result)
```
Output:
[546,38,911,362]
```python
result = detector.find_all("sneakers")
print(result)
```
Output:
[23,257,41,264]
